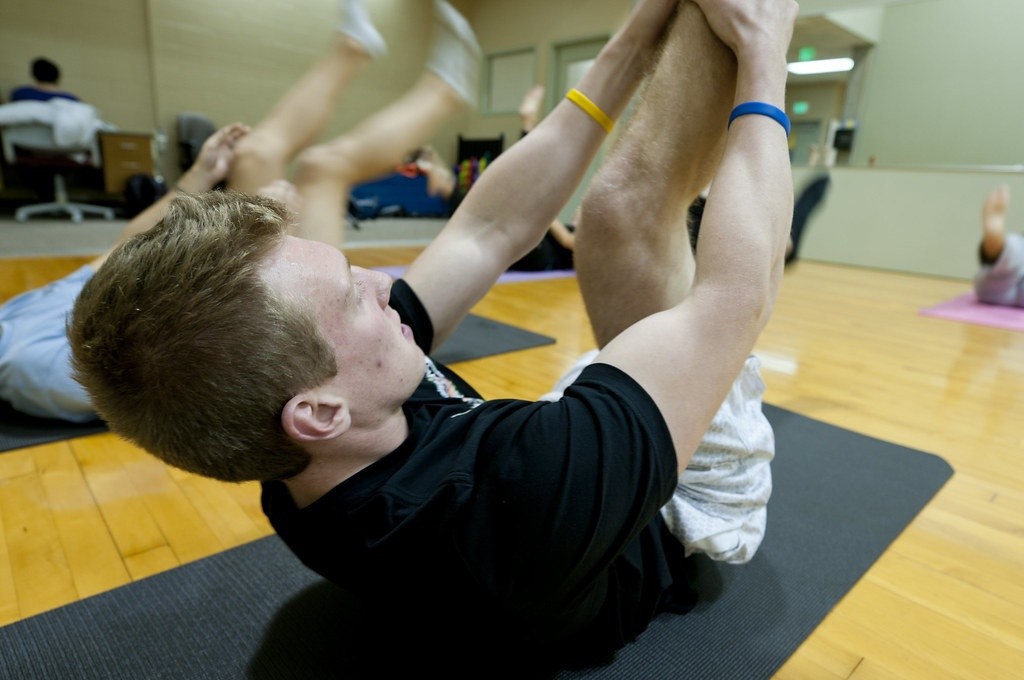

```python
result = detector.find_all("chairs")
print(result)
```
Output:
[8,140,116,223]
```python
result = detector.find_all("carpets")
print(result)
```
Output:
[370,262,578,282]
[924,289,1024,332]
[0,309,557,454]
[0,397,958,680]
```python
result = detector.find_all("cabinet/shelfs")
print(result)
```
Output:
[97,127,158,193]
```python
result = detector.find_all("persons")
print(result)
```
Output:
[418,86,581,273]
[687,140,837,273]
[11,56,99,201]
[972,183,1024,310]
[66,0,798,664]
[0,1,487,428]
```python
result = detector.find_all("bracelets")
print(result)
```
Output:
[727,102,791,136]
[565,88,613,134]
